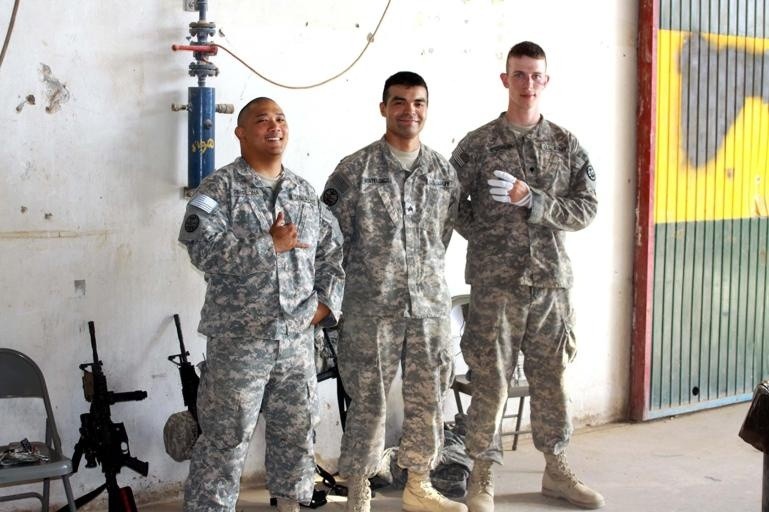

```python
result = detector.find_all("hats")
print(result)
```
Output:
[164,410,199,462]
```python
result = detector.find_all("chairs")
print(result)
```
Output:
[1,347,80,509]
[443,297,544,453]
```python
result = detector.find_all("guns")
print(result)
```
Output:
[316,329,352,433]
[72,321,149,512]
[168,314,199,433]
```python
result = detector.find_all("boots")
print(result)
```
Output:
[540,451,606,510]
[397,470,470,512]
[465,460,497,512]
[342,474,375,512]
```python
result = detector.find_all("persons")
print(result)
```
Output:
[320,71,469,511]
[179,97,346,511]
[449,41,606,512]
